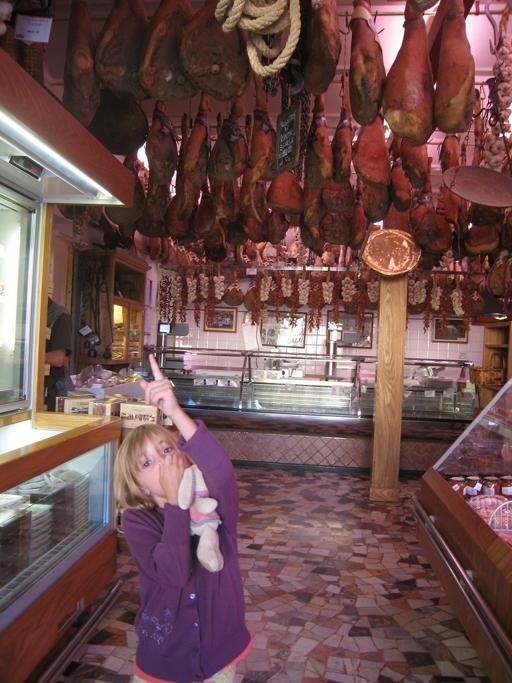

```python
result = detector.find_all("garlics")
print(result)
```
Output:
[174,235,466,316]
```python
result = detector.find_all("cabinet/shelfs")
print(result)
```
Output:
[430,381,512,545]
[146,346,481,424]
[1,410,119,631]
[0,183,48,411]
[482,322,512,388]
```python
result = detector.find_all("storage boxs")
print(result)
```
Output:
[55,392,172,426]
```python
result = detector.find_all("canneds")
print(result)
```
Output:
[449,475,512,502]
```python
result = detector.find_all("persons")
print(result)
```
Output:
[109,351,254,683]
[44,296,76,411]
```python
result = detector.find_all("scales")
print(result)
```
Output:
[165,323,189,369]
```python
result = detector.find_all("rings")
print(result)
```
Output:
[170,378,178,390]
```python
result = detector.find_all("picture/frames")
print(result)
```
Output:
[260,310,307,348]
[203,306,238,333]
[326,310,373,348]
[431,315,468,344]
